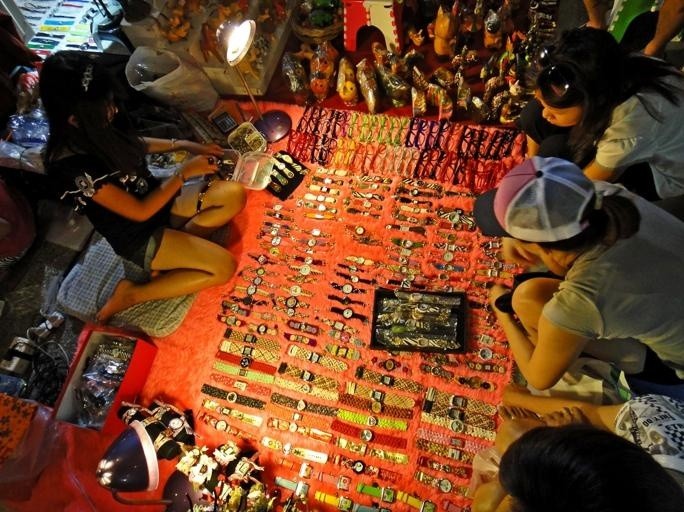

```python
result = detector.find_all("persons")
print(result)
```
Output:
[38,49,243,321]
[475,158,683,390]
[469,392,684,512]
[522,28,683,203]
[578,1,683,55]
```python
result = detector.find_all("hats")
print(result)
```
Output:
[472,153,604,244]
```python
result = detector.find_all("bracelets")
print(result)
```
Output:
[81,336,136,387]
[171,138,177,150]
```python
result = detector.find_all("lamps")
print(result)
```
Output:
[203,18,293,140]
[95,422,212,512]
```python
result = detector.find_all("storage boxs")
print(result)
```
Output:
[52,327,160,438]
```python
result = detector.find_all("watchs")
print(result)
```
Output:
[117,152,526,512]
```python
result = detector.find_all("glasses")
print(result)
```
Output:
[537,45,573,101]
